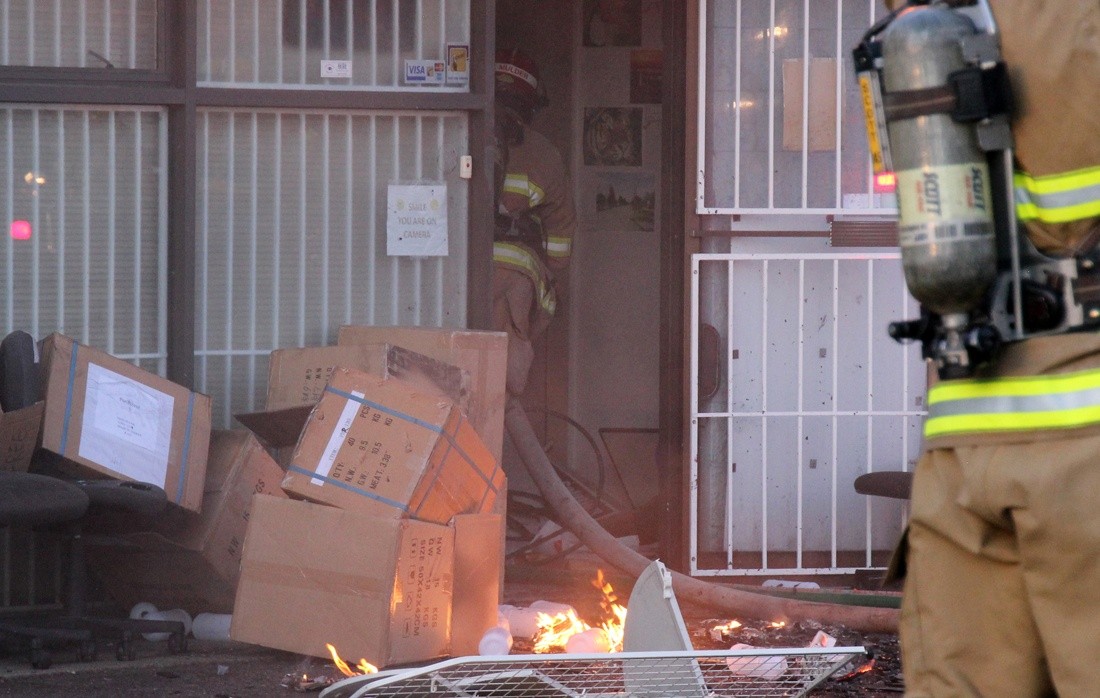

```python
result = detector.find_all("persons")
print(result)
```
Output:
[493,48,577,398]
[896,1,1100,697]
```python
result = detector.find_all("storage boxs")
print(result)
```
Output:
[0,325,509,670]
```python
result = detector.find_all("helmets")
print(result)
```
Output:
[496,47,548,108]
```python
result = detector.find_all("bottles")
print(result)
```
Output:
[479,627,514,656]
[139,609,192,641]
[761,579,821,590]
[191,612,233,642]
[726,642,790,680]
[565,627,611,654]
[499,598,580,638]
[129,601,157,619]
